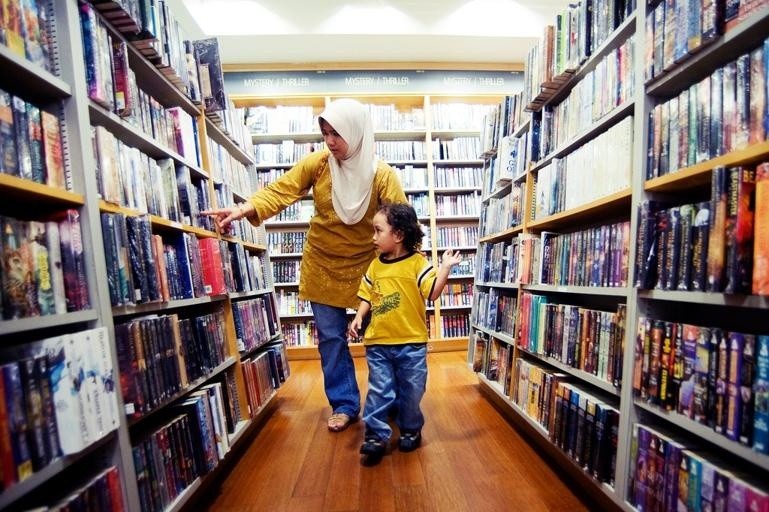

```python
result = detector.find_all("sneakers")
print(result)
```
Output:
[397,428,423,454]
[358,434,389,458]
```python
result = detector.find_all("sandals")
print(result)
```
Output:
[326,412,353,433]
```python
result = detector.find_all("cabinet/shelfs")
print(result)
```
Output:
[0,0,290,511]
[227,93,515,361]
[466,0,769,511]
